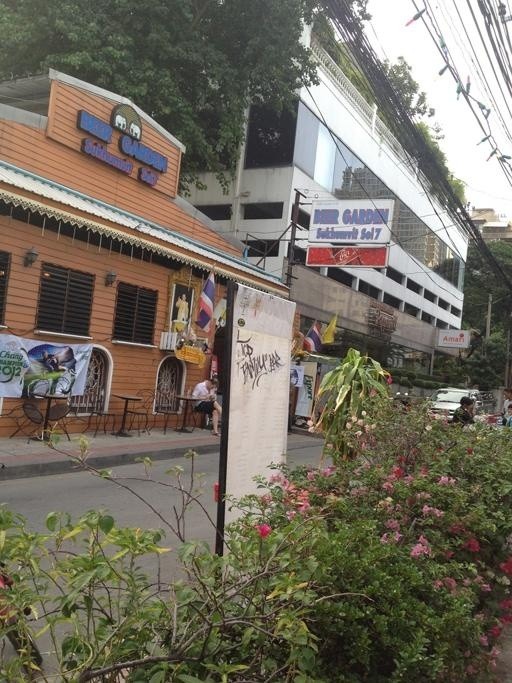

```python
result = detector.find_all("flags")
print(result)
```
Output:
[304,322,322,353]
[323,313,337,343]
[194,271,215,331]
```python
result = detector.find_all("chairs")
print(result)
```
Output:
[0,389,211,445]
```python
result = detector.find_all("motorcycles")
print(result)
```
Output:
[494,408,507,425]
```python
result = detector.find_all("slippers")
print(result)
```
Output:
[213,433,222,437]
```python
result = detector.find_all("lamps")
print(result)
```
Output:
[24,247,39,265]
[106,271,116,285]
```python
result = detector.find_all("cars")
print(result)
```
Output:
[424,388,484,423]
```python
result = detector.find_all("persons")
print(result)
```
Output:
[473,382,480,389]
[495,387,512,428]
[453,396,476,425]
[175,293,189,322]
[191,378,222,437]
